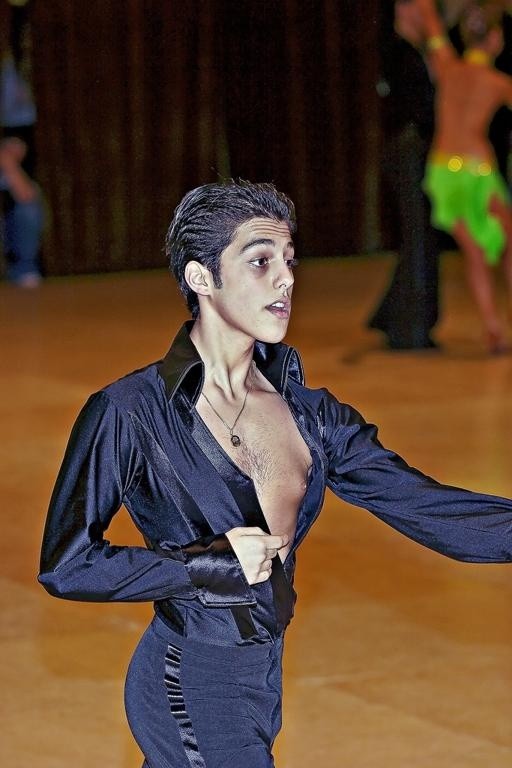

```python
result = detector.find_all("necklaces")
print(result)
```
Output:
[200,357,259,447]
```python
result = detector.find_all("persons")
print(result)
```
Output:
[35,176,512,767]
[1,62,44,291]
[365,0,440,351]
[392,0,510,359]
